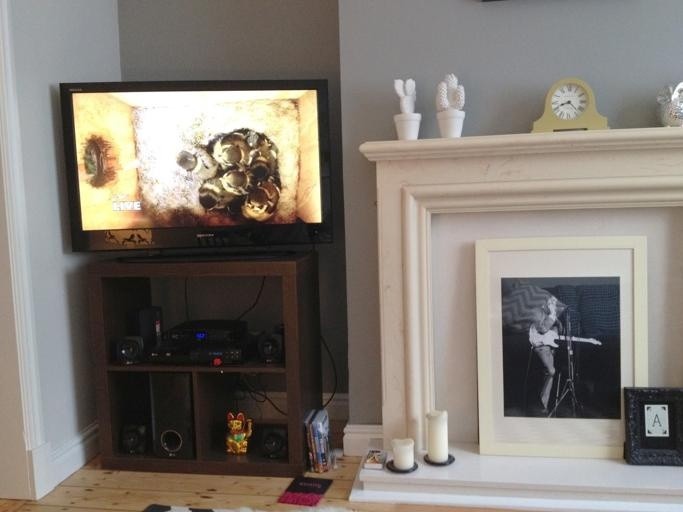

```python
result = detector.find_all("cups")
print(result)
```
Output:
[391,112,422,141]
[434,110,466,139]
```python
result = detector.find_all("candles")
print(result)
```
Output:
[388,408,449,470]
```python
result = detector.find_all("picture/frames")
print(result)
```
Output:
[473,235,648,466]
[623,386,682,465]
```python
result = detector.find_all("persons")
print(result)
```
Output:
[529,295,565,412]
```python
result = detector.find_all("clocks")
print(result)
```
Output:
[530,79,608,133]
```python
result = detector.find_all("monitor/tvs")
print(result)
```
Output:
[57,77,334,264]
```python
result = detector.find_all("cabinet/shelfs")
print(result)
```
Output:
[82,249,324,477]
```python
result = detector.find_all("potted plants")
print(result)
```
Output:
[391,70,468,141]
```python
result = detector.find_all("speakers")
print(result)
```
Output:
[121,424,148,457]
[260,425,288,458]
[255,323,283,366]
[118,333,146,364]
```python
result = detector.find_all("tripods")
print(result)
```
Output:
[544,308,587,418]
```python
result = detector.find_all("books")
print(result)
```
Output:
[305,407,332,474]
